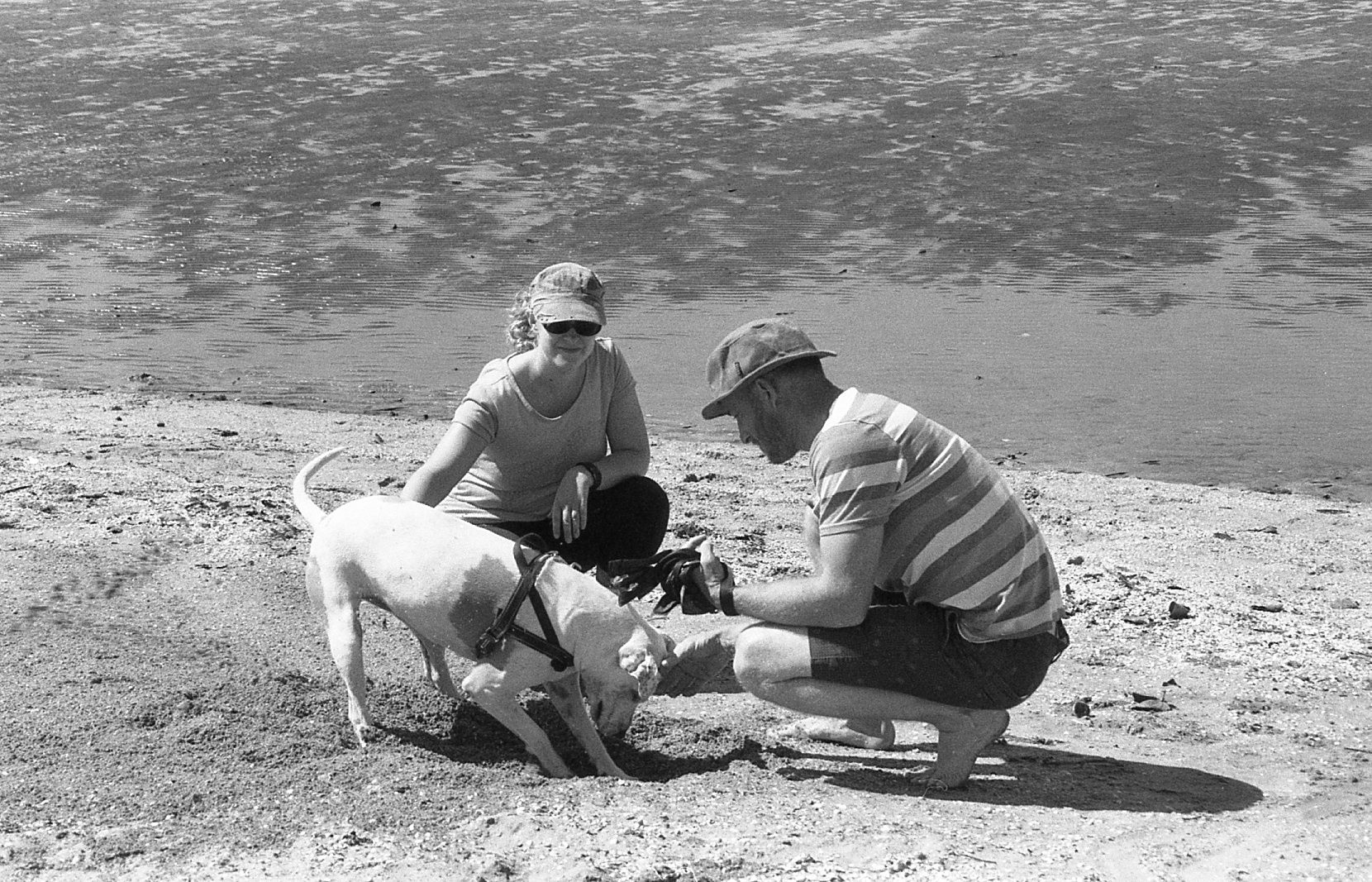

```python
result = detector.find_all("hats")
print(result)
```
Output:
[529,263,608,327]
[701,317,837,420]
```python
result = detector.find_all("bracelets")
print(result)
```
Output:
[576,461,602,490]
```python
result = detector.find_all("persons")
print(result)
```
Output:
[398,261,669,590]
[681,318,1069,787]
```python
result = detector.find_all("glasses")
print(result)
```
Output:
[542,321,602,336]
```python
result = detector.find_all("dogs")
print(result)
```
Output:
[292,445,671,780]
